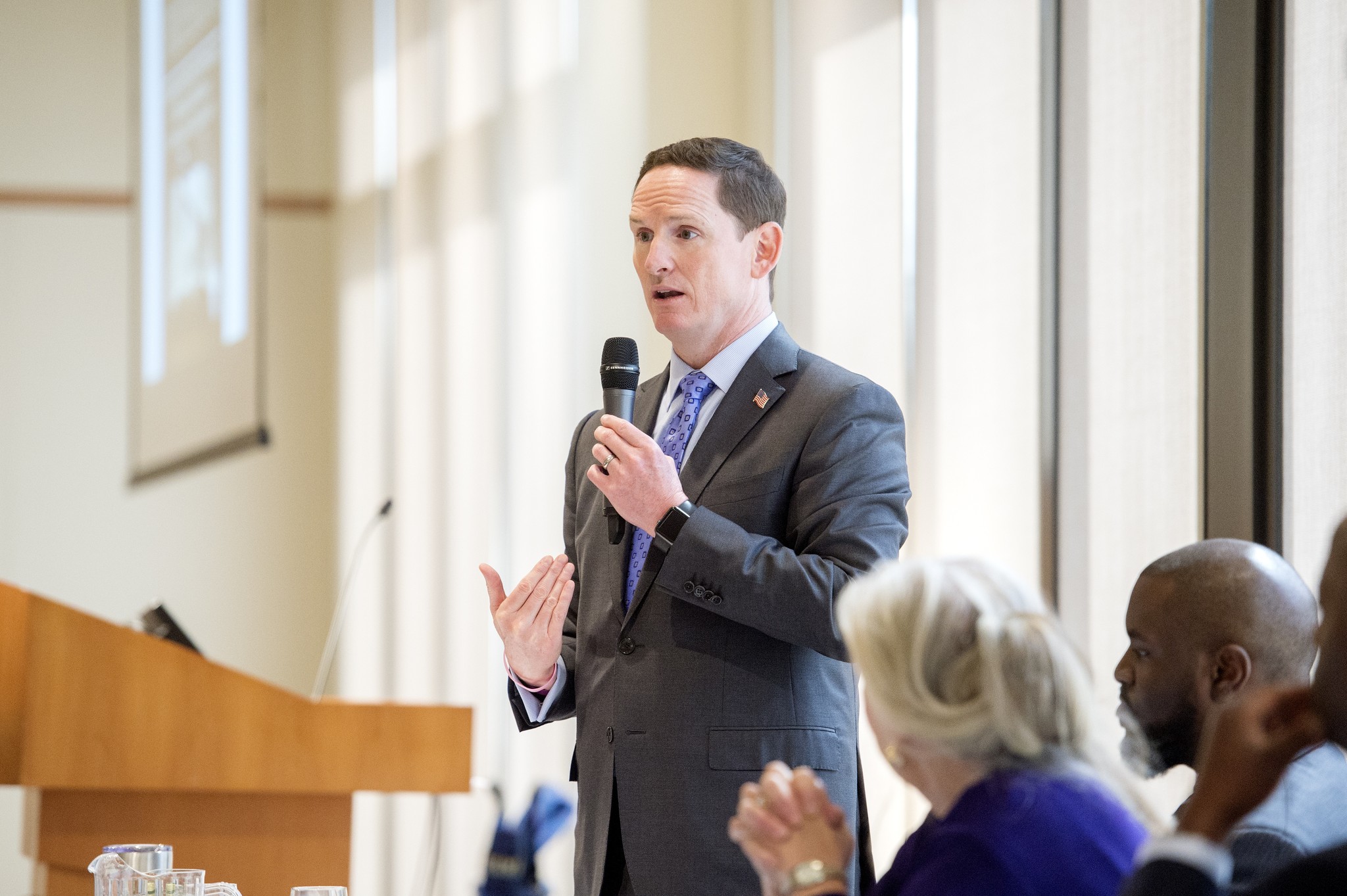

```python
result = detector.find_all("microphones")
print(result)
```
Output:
[600,338,640,545]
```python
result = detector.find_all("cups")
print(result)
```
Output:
[290,886,348,896]
[165,884,237,896]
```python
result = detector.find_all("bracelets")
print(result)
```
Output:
[504,647,559,693]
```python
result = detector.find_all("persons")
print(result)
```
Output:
[478,137,910,896]
[724,517,1347,896]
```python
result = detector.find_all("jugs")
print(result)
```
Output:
[87,852,243,896]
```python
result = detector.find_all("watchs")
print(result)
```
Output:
[770,859,848,896]
[652,500,698,555]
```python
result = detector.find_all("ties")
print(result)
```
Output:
[624,370,715,615]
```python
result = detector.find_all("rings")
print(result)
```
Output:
[603,454,617,470]
